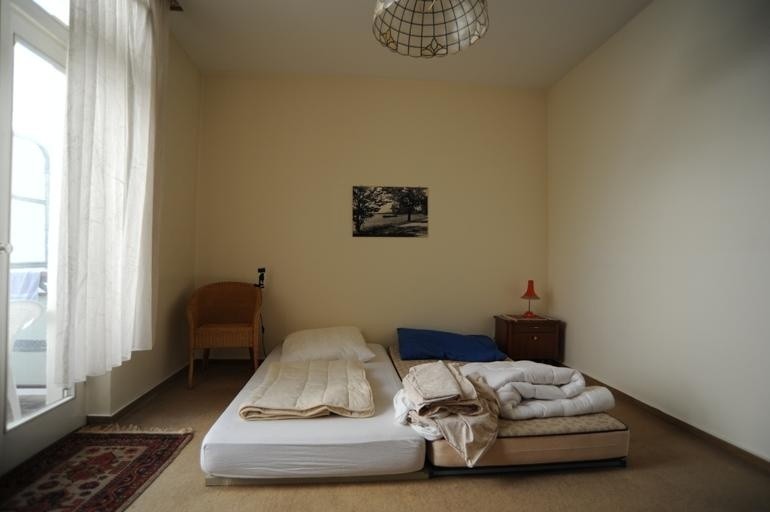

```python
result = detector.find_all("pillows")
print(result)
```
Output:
[399,324,508,362]
[282,326,376,364]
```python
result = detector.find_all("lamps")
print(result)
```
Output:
[371,0,490,58]
[521,280,541,318]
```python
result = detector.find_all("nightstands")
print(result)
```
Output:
[493,313,566,365]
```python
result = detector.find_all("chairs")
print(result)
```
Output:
[185,280,265,389]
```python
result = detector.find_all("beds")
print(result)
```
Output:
[201,342,428,488]
[388,342,630,477]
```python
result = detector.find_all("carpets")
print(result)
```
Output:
[0,424,194,512]
